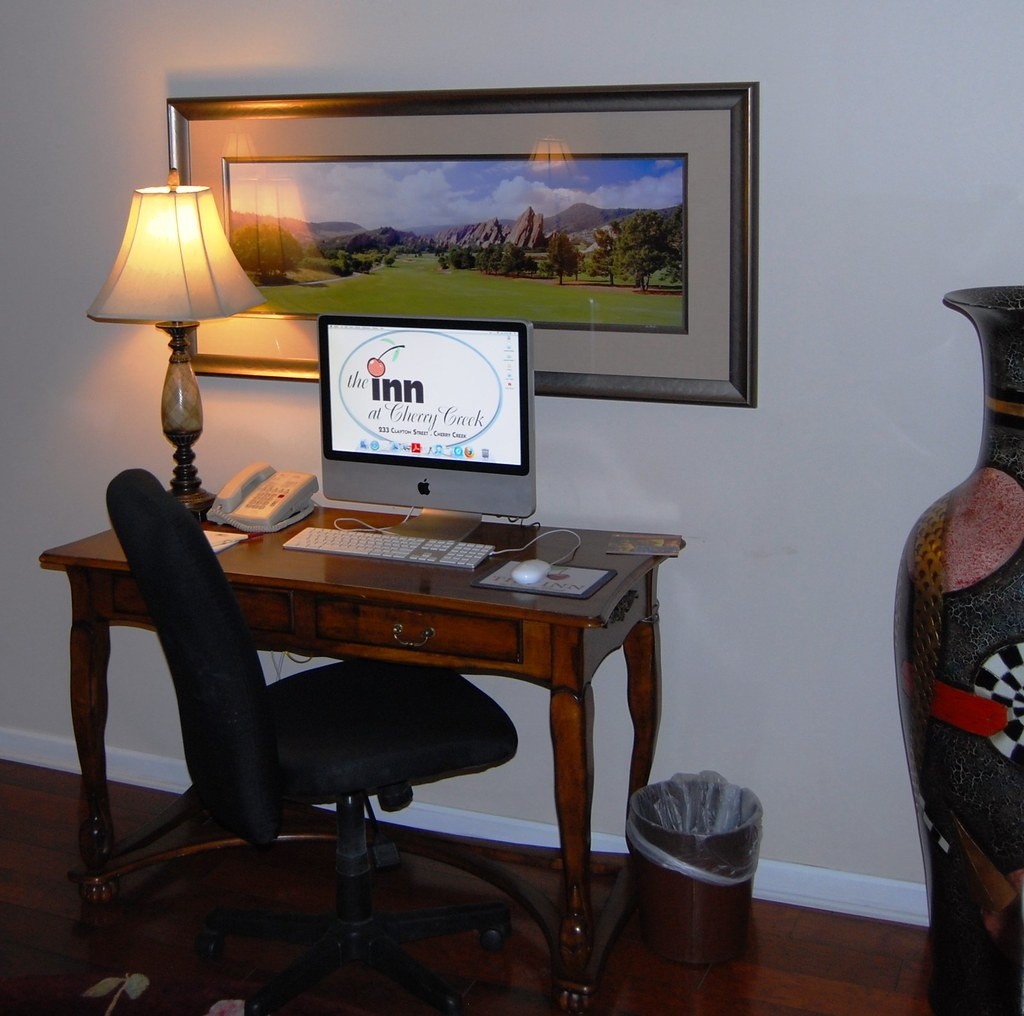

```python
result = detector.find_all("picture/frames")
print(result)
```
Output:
[165,81,761,410]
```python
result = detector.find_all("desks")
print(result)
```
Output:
[39,507,685,1016]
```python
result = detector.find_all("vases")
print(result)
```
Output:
[894,284,1024,1016]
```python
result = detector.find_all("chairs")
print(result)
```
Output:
[106,466,519,1016]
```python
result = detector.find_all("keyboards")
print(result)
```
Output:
[283,527,495,569]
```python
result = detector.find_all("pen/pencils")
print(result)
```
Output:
[212,531,264,548]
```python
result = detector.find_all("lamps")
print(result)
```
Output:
[87,168,269,524]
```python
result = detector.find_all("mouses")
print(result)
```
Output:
[511,559,552,583]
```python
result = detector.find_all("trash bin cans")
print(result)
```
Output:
[626,785,764,969]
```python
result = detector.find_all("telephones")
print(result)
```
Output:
[205,462,320,530]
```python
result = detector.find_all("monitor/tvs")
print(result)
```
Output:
[319,313,536,538]
